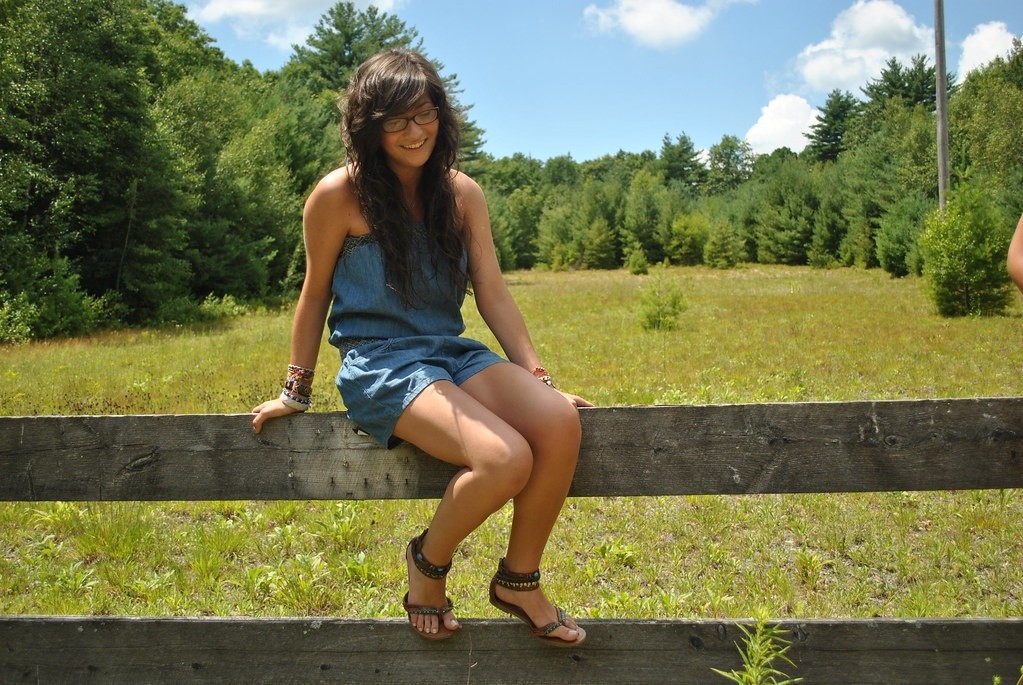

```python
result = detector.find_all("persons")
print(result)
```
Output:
[1005,213,1023,291]
[252,49,596,646]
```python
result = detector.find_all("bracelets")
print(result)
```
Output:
[281,364,314,412]
[531,367,554,388]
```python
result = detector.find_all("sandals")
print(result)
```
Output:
[489,556,587,648]
[402,527,458,641]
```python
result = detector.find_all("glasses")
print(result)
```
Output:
[378,106,439,134]
